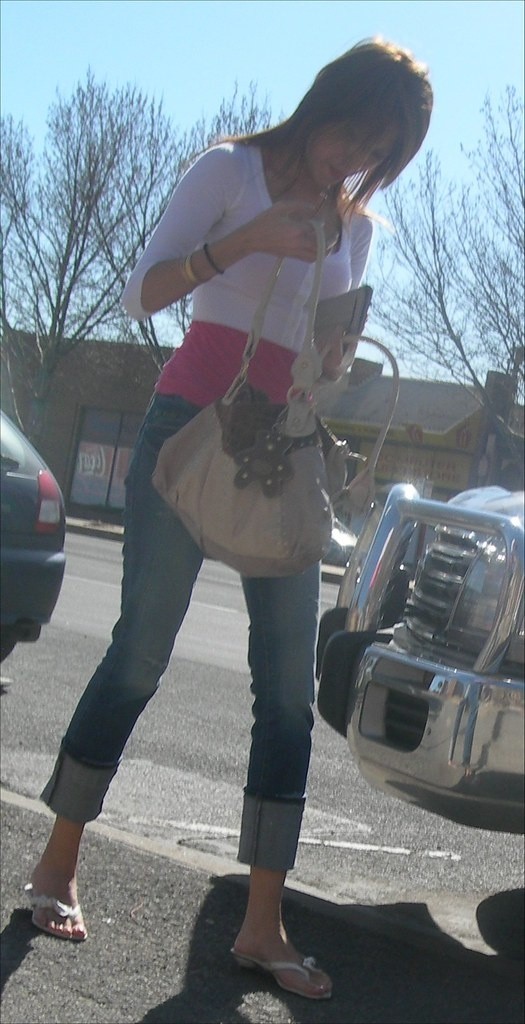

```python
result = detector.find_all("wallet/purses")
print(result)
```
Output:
[312,286,373,347]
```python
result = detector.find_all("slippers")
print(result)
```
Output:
[223,943,332,1001]
[24,882,88,940]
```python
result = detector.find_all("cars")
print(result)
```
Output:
[321,516,359,566]
[1,410,65,663]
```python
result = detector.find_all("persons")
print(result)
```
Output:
[27,44,433,999]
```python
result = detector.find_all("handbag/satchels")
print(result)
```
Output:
[152,218,400,578]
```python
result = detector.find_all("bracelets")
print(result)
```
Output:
[184,254,200,286]
[204,244,226,275]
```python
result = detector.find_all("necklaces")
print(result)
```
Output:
[311,184,330,218]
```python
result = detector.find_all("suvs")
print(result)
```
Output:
[316,482,525,835]
